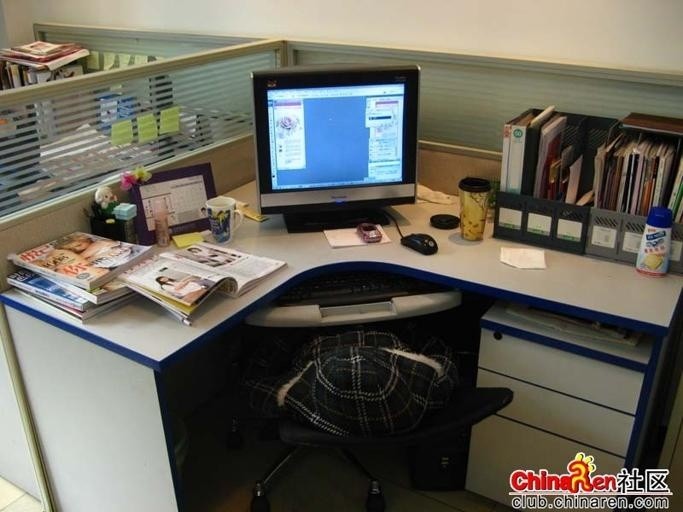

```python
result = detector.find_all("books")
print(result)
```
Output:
[6,228,157,323]
[498,104,683,226]
[119,242,288,327]
[1,41,97,143]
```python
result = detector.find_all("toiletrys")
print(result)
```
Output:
[636,207,673,276]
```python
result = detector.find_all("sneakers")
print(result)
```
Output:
[408,458,466,492]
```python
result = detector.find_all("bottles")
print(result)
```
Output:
[153,199,171,248]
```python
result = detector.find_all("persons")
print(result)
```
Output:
[152,274,208,298]
[201,207,207,217]
[186,246,229,266]
[94,184,119,212]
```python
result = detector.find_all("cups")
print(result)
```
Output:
[459,177,491,243]
[206,198,243,245]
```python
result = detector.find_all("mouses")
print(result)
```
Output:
[403,231,440,256]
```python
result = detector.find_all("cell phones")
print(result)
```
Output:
[355,222,382,241]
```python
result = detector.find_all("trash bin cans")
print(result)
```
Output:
[409,350,478,491]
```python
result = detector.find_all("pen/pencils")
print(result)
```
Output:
[82,202,103,220]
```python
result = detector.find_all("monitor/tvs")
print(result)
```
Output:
[251,64,419,235]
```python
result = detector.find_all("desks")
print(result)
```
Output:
[0,177,683,512]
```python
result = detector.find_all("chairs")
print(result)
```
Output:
[232,353,512,511]
